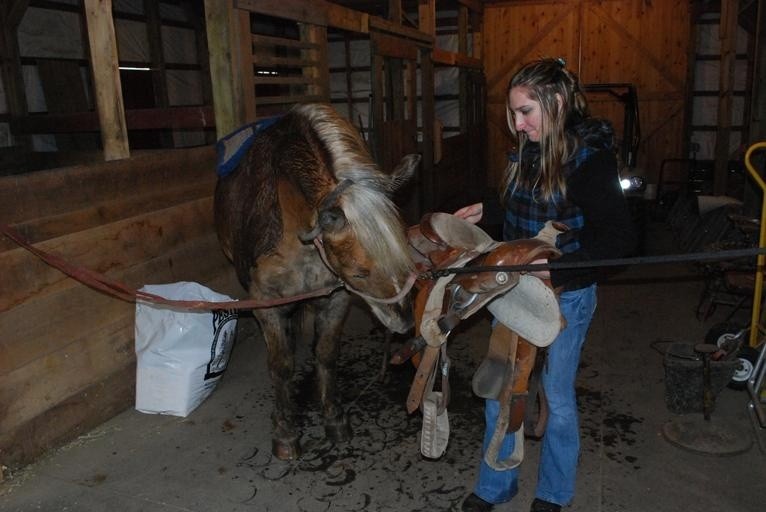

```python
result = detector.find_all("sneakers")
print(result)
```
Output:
[530,498,561,512]
[461,492,494,512]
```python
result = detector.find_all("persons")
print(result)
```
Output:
[454,54,634,511]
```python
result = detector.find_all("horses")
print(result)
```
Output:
[213,102,421,461]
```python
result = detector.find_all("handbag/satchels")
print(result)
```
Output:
[132,281,239,418]
